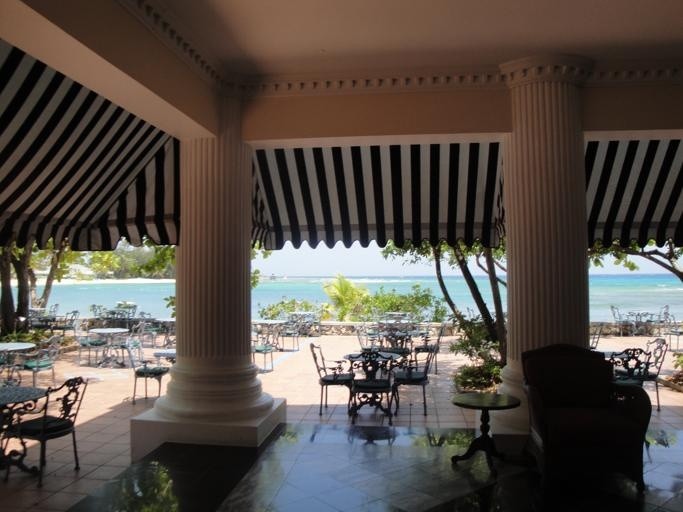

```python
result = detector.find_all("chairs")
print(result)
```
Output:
[250,302,321,374]
[591,302,683,413]
[309,312,446,427]
[0,303,175,488]
[514,342,652,509]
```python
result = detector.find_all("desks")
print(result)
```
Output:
[450,393,521,477]
